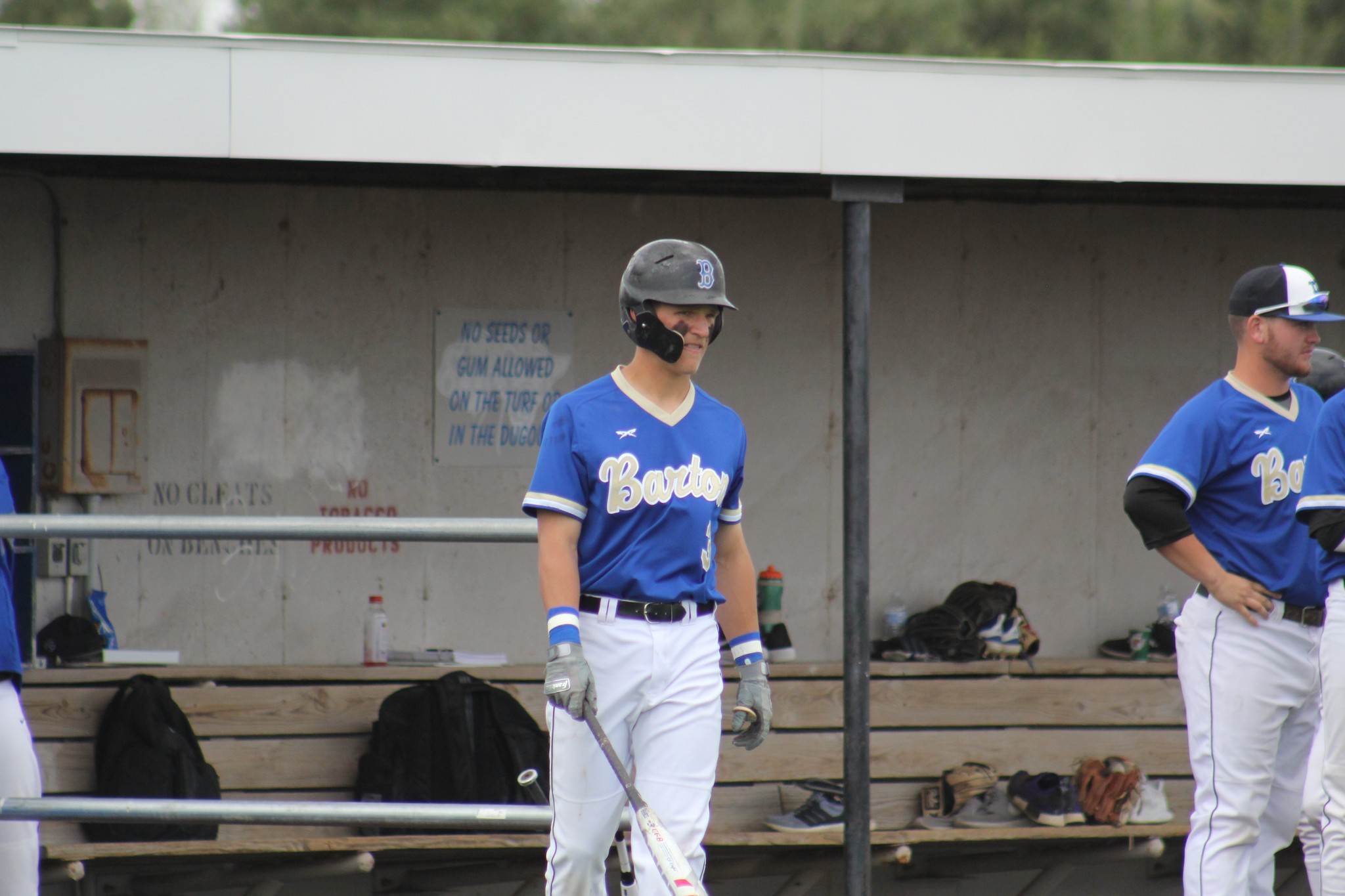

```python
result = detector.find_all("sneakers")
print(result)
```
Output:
[1010,770,1065,827]
[913,795,982,829]
[950,781,1040,828]
[763,773,874,833]
[1060,782,1086,824]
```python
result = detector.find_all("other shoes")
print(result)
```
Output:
[1129,772,1174,824]
[1100,637,1133,658]
[1145,620,1176,660]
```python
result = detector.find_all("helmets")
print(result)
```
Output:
[621,234,733,358]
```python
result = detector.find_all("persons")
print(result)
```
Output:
[1122,261,1345,896]
[522,239,773,896]
[0,457,42,896]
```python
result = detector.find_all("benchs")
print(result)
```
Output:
[11,654,1195,887]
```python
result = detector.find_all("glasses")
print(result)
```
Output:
[1254,291,1331,316]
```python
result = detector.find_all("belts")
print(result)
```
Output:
[1197,583,1327,628]
[579,594,715,624]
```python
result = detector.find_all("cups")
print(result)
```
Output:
[1128,628,1152,661]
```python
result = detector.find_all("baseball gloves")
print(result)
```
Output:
[1075,757,1143,828]
[918,762,999,819]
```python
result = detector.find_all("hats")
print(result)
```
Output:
[1229,262,1345,322]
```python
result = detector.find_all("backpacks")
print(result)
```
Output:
[354,670,550,835]
[89,673,221,841]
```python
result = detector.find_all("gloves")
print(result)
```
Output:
[731,658,773,750]
[544,641,597,721]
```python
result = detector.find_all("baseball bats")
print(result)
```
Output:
[581,700,709,896]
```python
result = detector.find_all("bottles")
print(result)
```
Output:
[883,591,908,640]
[1158,580,1181,625]
[363,594,390,666]
[757,564,783,638]
